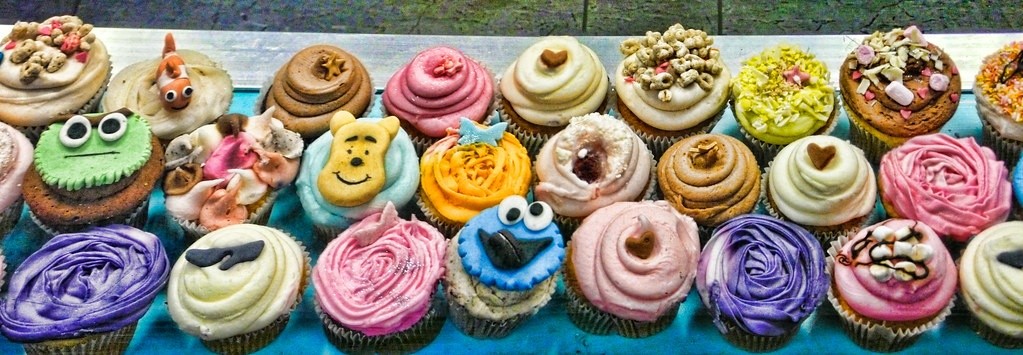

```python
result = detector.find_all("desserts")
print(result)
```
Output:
[0,15,1023,355]
